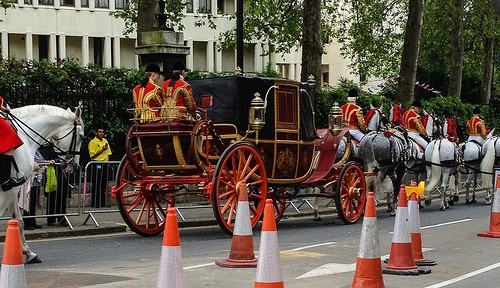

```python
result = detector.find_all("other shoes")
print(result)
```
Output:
[34,223,41,229]
[24,223,34,230]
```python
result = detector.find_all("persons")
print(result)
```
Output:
[44,143,75,227]
[133,63,164,125]
[22,149,56,231]
[466,107,487,146]
[401,100,428,149]
[88,126,112,208]
[0,95,28,192]
[160,60,204,152]
[340,89,369,142]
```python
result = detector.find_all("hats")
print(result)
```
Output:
[473,107,480,114]
[172,61,186,70]
[145,63,160,72]
[348,88,358,97]
[411,99,423,108]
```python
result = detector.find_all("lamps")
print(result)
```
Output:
[249,92,266,145]
[328,101,342,148]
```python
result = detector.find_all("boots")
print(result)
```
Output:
[0,155,27,192]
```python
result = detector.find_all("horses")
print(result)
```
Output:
[0,104,84,264]
[313,129,499,220]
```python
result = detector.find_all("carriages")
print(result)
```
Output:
[114,66,500,237]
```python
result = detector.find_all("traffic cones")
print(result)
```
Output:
[215,180,258,268]
[0,219,27,288]
[351,192,386,288]
[254,199,284,288]
[477,173,500,239]
[157,207,184,288]
[384,181,437,266]
[383,184,431,275]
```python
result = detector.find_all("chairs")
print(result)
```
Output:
[126,108,216,176]
